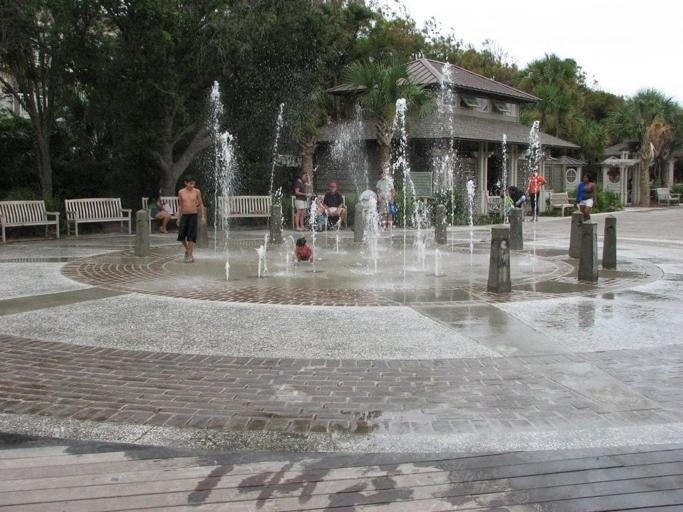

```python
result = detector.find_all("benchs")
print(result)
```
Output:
[607,182,620,199]
[656,188,681,207]
[488,196,514,214]
[549,192,576,216]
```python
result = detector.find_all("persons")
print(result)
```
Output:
[526,169,547,222]
[175,175,206,263]
[375,166,396,231]
[315,182,349,232]
[507,185,525,222]
[575,173,598,222]
[294,236,312,264]
[147,184,170,234]
[292,172,312,232]
